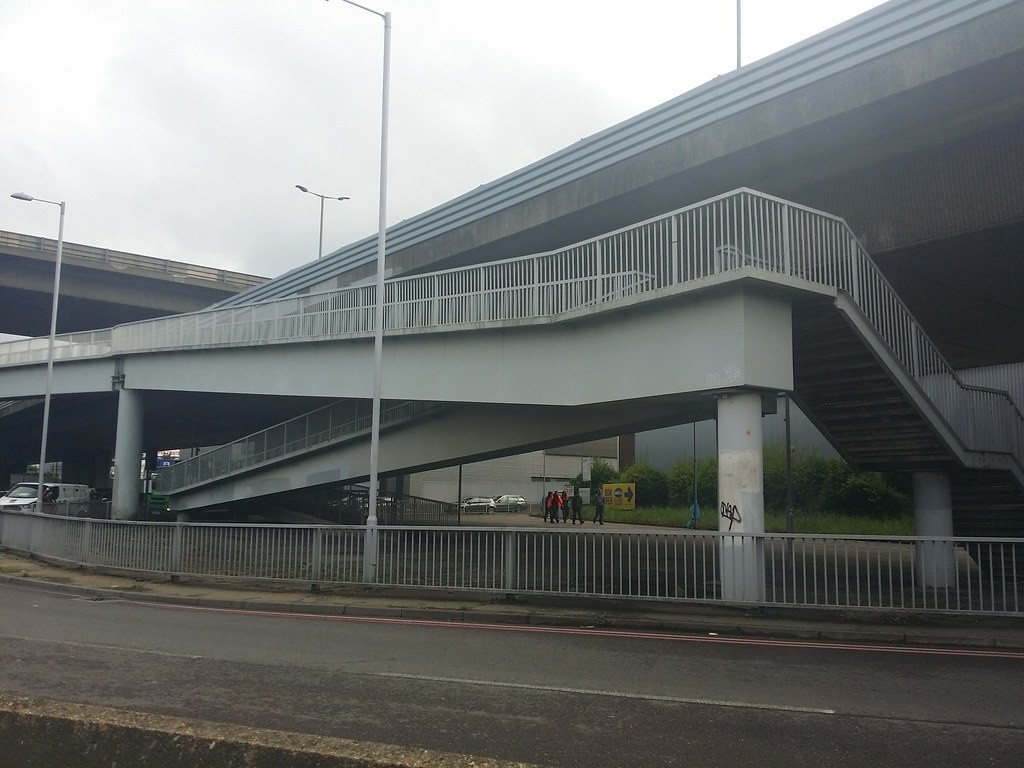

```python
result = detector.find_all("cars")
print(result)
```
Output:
[330,495,400,515]
[490,495,529,513]
[446,496,496,515]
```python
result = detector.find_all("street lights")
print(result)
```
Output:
[325,0,392,581]
[296,183,351,260]
[10,193,66,516]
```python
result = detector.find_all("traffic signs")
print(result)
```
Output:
[602,484,635,510]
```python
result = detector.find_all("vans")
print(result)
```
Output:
[0,483,90,517]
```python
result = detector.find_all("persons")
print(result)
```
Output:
[544,491,570,524]
[593,495,605,525]
[571,491,585,525]
